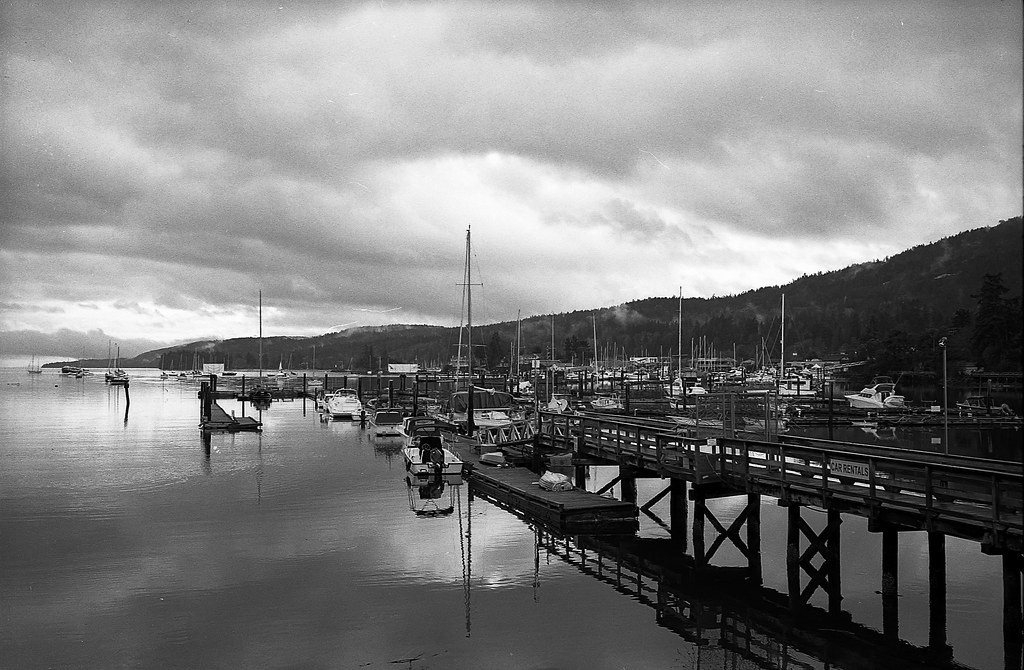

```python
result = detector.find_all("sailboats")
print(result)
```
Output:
[25,353,42,374]
[503,280,906,436]
[105,339,129,385]
[158,347,238,379]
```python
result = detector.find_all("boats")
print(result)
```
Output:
[367,410,405,437]
[394,415,464,476]
[327,387,363,421]
